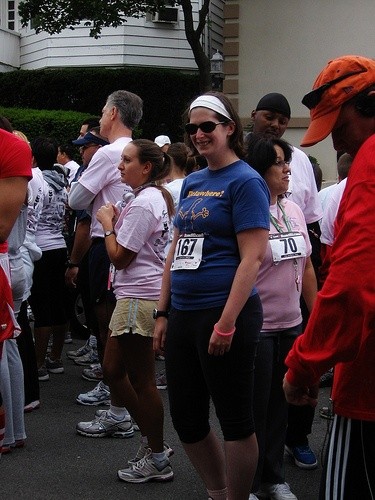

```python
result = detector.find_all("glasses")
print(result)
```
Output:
[272,161,291,168]
[301,70,367,110]
[184,121,225,135]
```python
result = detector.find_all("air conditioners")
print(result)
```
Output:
[151,7,179,24]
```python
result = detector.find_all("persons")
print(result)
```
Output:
[239,131,317,500]
[14,134,82,410]
[1,187,25,453]
[0,128,32,448]
[281,54,375,500]
[97,141,173,483]
[307,152,354,419]
[156,133,193,215]
[246,90,324,277]
[66,91,144,439]
[153,92,270,500]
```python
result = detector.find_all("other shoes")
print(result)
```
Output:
[319,373,334,387]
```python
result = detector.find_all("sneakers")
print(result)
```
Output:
[45,357,64,373]
[38,365,49,380]
[67,339,97,358]
[95,410,140,430]
[82,368,103,380]
[319,406,334,419]
[76,380,111,405]
[117,448,174,483]
[64,333,72,342]
[285,443,317,469]
[127,440,174,464]
[76,411,134,437]
[74,350,100,365]
[267,481,298,500]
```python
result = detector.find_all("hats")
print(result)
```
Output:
[256,93,291,120]
[154,135,171,147]
[300,55,375,147]
[72,132,110,146]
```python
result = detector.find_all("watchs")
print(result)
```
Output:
[104,230,115,238]
[66,259,80,268]
[153,308,167,319]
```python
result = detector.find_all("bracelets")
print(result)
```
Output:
[215,323,236,336]
